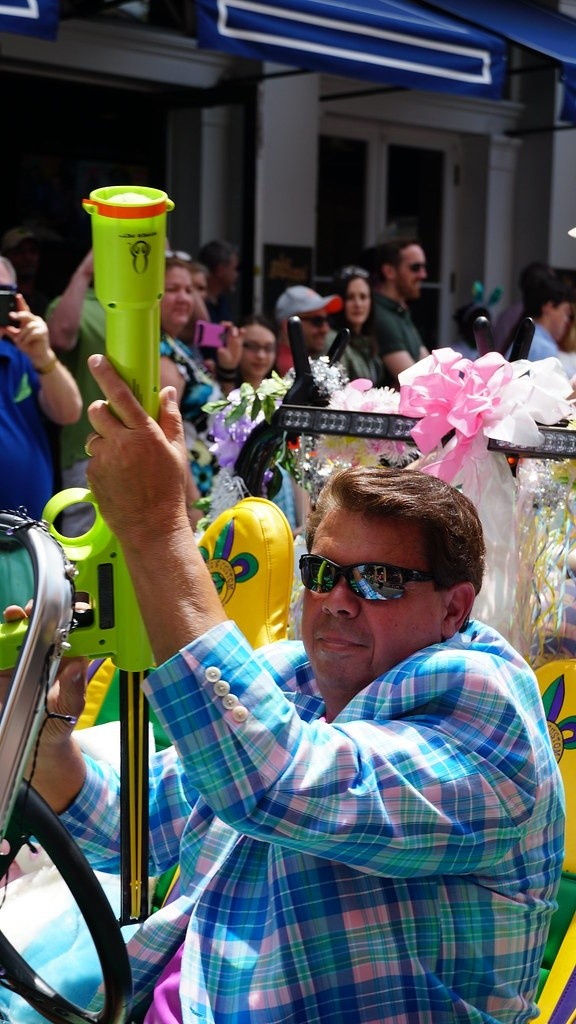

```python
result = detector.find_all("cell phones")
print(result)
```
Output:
[193,320,227,348]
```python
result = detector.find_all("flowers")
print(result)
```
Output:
[187,347,576,529]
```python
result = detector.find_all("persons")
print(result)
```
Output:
[1,352,567,1023]
[159,238,436,537]
[351,566,388,600]
[0,255,81,521]
[44,247,109,539]
[448,262,576,402]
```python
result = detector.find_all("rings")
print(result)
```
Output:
[84,435,102,457]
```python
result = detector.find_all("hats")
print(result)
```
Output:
[275,286,343,323]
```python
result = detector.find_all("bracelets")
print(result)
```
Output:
[34,355,58,375]
[217,365,237,383]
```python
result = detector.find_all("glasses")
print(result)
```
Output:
[298,552,431,604]
[395,262,429,274]
[242,339,277,356]
[340,265,370,281]
[164,247,192,263]
[298,313,335,328]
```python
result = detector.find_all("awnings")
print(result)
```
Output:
[196,0,508,106]
[0,0,59,43]
[434,0,576,137]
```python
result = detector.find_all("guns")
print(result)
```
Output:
[0,184,177,928]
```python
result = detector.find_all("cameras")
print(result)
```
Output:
[0,292,19,328]
